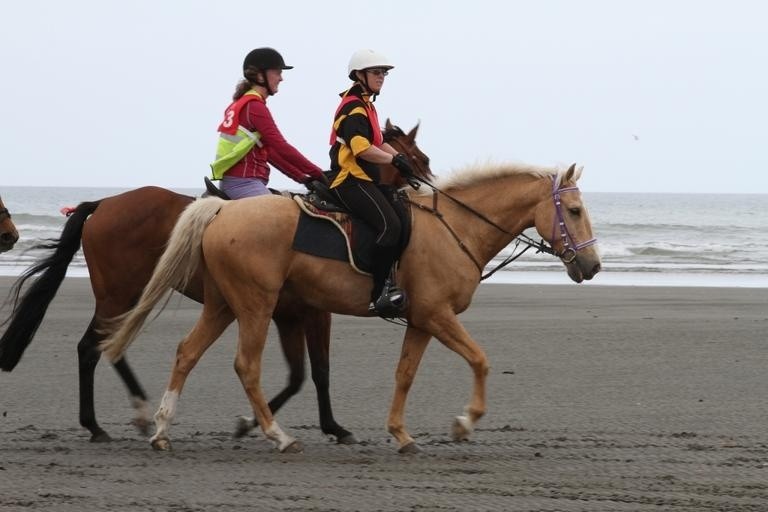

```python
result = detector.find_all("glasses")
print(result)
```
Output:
[366,69,389,76]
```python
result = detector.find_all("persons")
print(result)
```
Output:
[336,46,414,310]
[210,48,331,198]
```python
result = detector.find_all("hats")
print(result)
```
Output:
[243,48,293,70]
[348,49,395,81]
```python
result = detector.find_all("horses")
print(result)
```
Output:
[0,117,440,444]
[0,197,19,256]
[96,163,601,456]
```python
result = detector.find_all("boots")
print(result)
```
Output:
[368,243,404,313]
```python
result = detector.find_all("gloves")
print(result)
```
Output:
[299,176,316,190]
[391,154,413,178]
[317,175,330,187]
